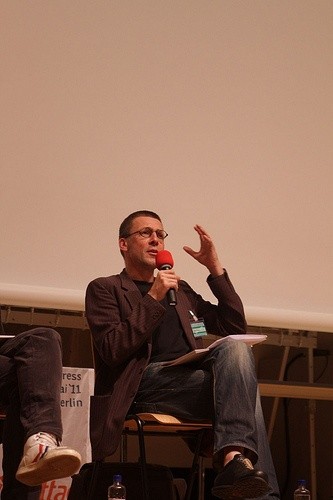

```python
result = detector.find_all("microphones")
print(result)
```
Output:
[156,250,177,306]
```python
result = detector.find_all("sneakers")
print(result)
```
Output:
[14,431,81,487]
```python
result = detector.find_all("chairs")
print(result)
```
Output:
[118,400,214,499]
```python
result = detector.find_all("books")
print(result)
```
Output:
[206,334,270,350]
[158,347,209,369]
[0,334,16,347]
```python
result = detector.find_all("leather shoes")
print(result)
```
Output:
[210,454,268,500]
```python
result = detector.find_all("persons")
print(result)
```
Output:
[85,211,280,500]
[0,327,82,500]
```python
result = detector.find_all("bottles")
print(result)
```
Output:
[108,475,126,500]
[294,480,310,500]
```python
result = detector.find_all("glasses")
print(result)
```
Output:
[123,229,169,240]
